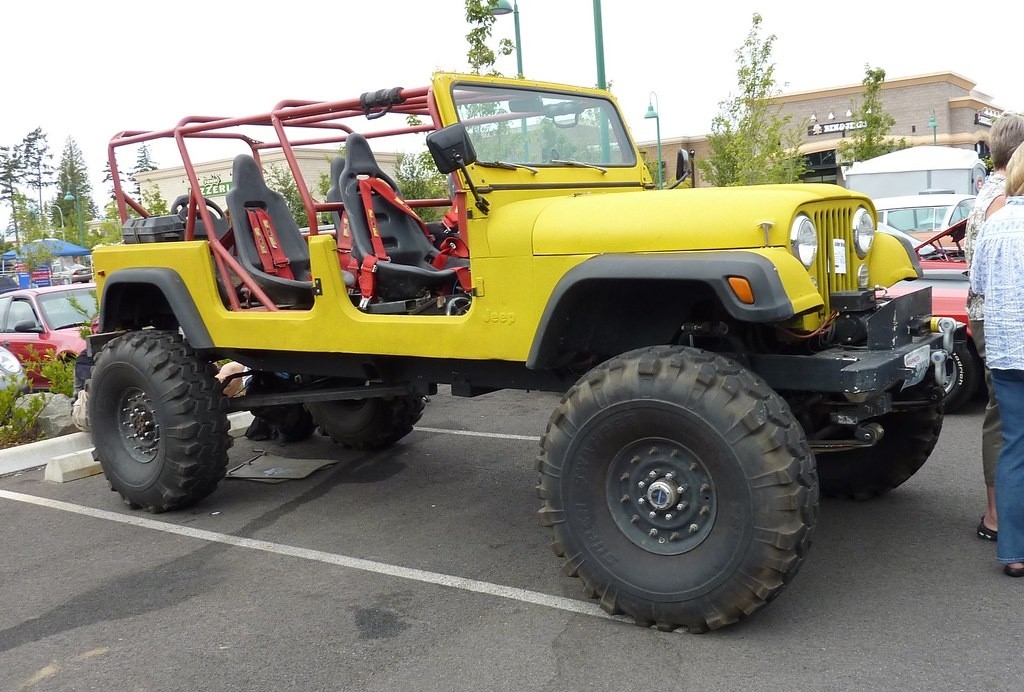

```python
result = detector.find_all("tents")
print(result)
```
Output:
[2,238,93,274]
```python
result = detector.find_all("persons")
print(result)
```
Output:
[70,316,99,432]
[964,107,1024,576]
[214,361,318,442]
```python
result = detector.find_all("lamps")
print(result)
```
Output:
[827,108,835,119]
[811,110,818,122]
[977,107,1001,118]
[846,106,853,118]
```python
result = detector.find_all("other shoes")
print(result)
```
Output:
[1004,562,1024,576]
[977,514,998,541]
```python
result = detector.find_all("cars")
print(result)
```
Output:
[0,283,100,395]
[52,263,92,285]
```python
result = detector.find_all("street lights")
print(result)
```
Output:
[488,0,531,166]
[644,91,663,191]
[62,183,88,268]
[927,107,938,145]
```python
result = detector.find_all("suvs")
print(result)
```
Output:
[73,72,970,635]
[868,191,985,417]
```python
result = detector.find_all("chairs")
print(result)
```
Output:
[224,154,314,306]
[338,131,471,304]
[326,157,345,243]
